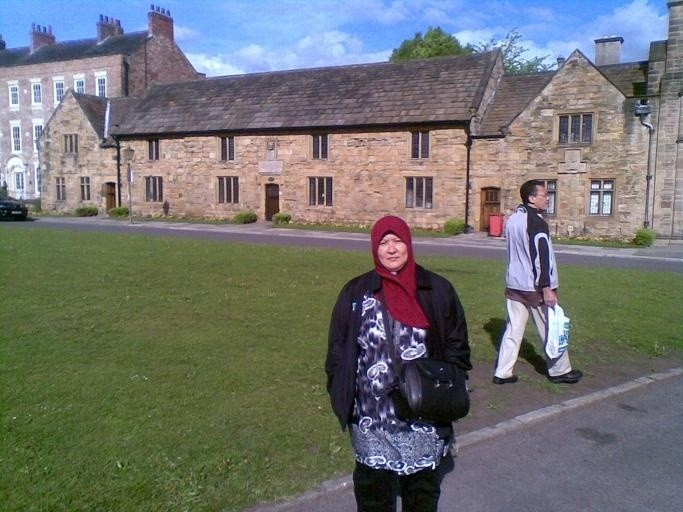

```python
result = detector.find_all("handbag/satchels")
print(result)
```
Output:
[389,357,467,423]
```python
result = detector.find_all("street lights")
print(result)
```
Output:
[123,144,136,223]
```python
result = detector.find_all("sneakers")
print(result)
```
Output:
[550,369,583,383]
[493,373,518,384]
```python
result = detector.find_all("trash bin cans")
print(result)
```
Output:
[488,213,505,237]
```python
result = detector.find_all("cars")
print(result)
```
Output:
[0,196,28,222]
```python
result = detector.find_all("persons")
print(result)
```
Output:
[325,215,471,512]
[492,179,583,385]
